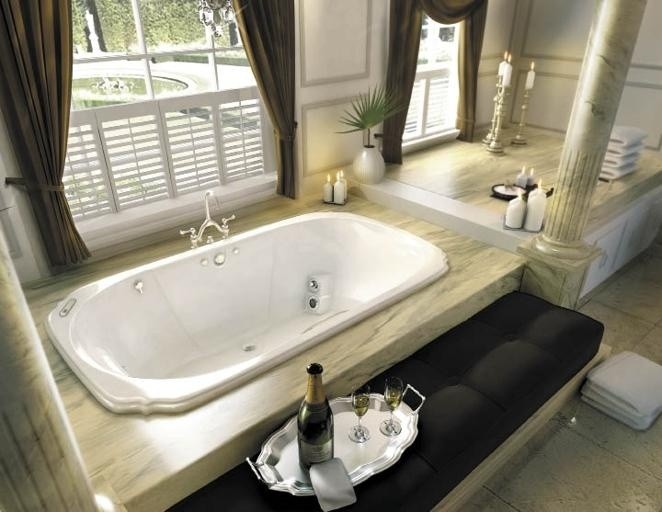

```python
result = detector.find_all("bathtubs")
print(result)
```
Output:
[43,210,449,415]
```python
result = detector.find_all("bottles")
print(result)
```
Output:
[297,363,336,470]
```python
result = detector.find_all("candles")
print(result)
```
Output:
[524,61,536,91]
[503,166,546,233]
[323,169,347,206]
[497,50,508,76]
[502,53,513,86]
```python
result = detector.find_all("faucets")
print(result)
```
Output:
[179,188,238,250]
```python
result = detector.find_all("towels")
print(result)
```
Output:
[599,125,648,180]
[579,351,662,432]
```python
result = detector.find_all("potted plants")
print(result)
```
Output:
[333,83,409,185]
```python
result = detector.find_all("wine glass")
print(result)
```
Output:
[380,376,404,437]
[348,382,371,444]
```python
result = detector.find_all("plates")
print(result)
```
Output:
[493,182,528,199]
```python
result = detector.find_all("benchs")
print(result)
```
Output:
[168,290,612,512]
